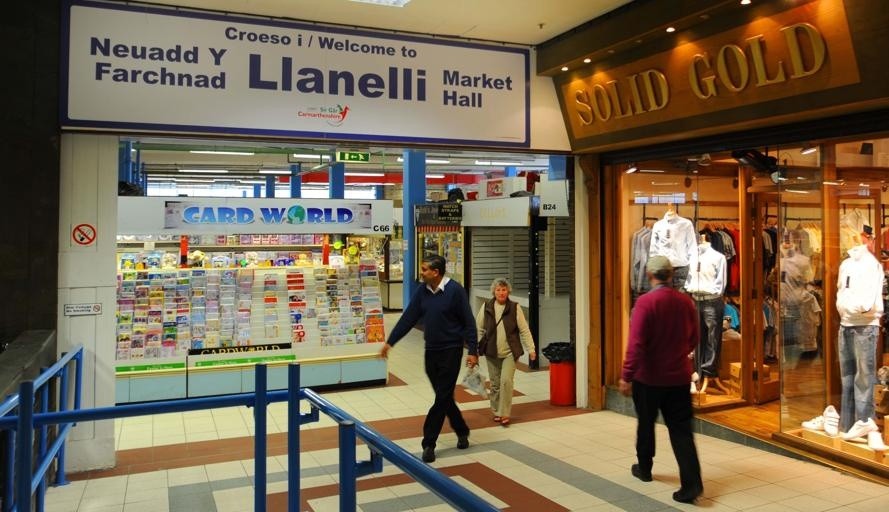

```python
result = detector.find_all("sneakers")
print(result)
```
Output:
[844,419,879,441]
[673,483,704,502]
[631,464,654,483]
[802,416,824,431]
[823,405,841,437]
[867,431,884,449]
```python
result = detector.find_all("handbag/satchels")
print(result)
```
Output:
[478,332,488,356]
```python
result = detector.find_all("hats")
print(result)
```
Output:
[646,257,674,273]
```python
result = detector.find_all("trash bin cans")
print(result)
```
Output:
[542,343,576,406]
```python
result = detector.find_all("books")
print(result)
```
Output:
[117,235,385,362]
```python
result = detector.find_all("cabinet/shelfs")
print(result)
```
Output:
[115,264,389,404]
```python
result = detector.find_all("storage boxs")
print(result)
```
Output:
[725,360,780,403]
[871,383,889,452]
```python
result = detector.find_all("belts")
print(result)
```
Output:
[693,294,720,300]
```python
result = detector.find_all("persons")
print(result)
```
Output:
[379,255,479,462]
[692,243,728,380]
[469,277,538,426]
[619,255,704,503]
[836,247,884,441]
[651,210,696,290]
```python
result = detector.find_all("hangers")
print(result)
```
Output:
[796,218,821,230]
[646,218,656,228]
[698,221,747,231]
[762,220,782,227]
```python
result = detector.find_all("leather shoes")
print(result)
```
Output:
[457,427,470,449]
[422,447,436,463]
[494,417,501,422]
[500,417,511,425]
